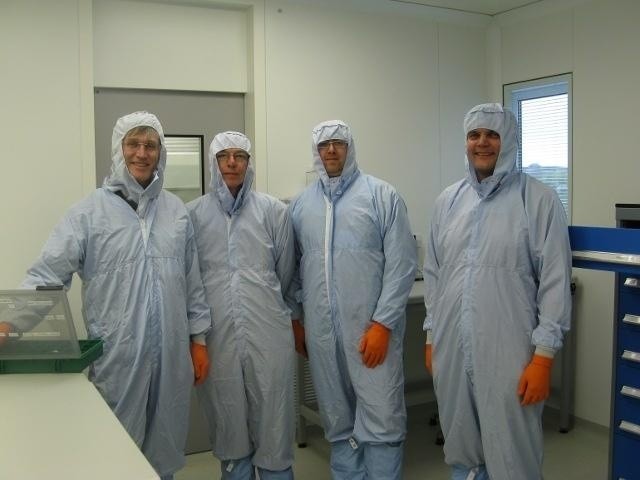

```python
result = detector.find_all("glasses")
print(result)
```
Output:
[127,141,156,151]
[217,153,247,161]
[318,141,344,148]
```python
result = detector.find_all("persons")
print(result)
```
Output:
[0,110,212,479]
[422,102,574,480]
[183,130,298,480]
[287,119,419,479]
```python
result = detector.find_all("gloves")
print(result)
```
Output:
[291,319,307,357]
[425,344,432,374]
[517,355,551,405]
[0,323,10,343]
[359,322,389,367]
[191,342,210,384]
